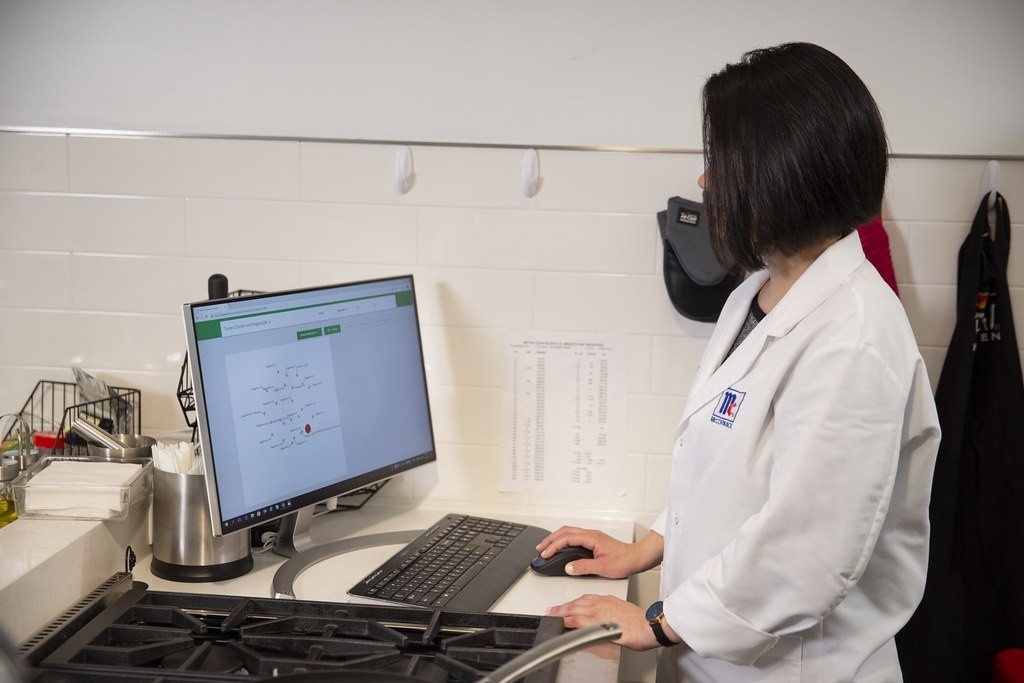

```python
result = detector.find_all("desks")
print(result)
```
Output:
[131,499,640,683]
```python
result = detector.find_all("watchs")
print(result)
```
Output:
[645,601,680,648]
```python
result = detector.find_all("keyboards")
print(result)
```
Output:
[344,513,552,610]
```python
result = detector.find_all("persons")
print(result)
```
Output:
[535,42,943,683]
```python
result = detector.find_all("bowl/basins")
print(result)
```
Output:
[88,433,156,458]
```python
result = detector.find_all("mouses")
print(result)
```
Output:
[530,545,598,577]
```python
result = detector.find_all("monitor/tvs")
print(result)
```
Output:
[184,274,435,600]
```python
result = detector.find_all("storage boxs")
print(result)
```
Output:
[9,451,157,522]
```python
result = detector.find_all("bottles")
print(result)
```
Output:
[0,429,87,529]
